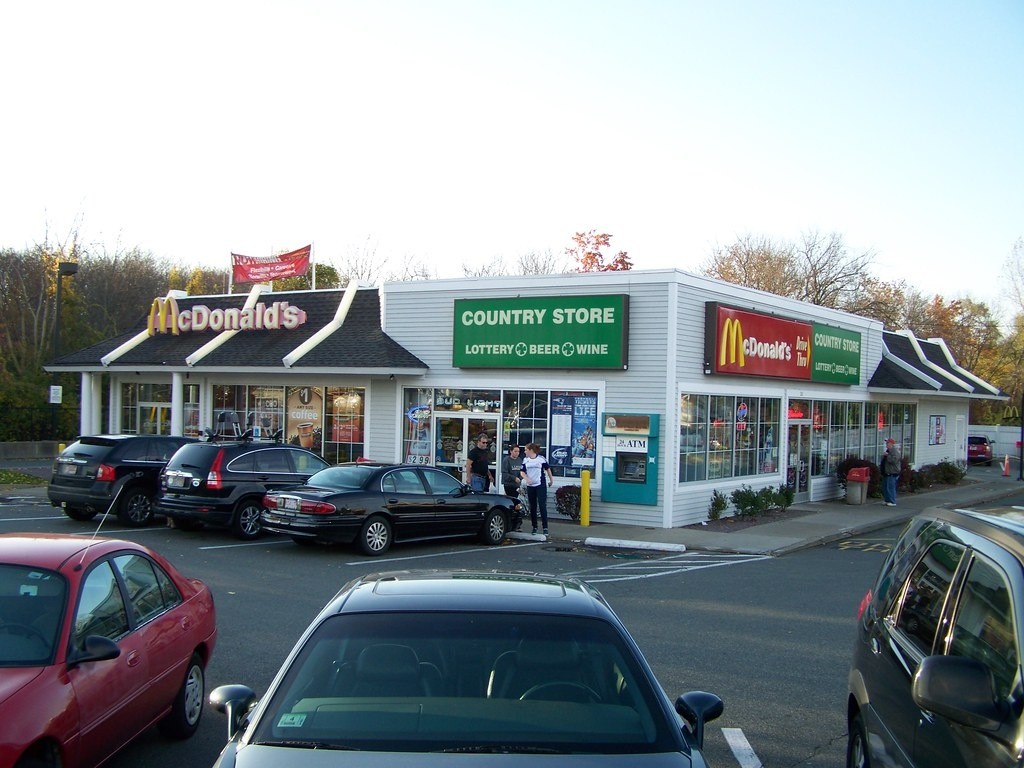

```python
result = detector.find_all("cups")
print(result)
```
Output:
[297,422,314,448]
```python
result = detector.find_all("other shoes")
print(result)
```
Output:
[881,501,897,507]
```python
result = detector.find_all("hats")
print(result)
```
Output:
[885,439,895,444]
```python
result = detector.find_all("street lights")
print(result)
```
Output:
[50,262,80,439]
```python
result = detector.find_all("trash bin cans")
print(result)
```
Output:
[845,467,870,504]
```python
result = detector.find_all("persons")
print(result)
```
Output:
[880,437,901,506]
[520,443,554,536]
[465,433,495,492]
[501,445,522,498]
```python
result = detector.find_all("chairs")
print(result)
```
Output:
[328,643,447,697]
[21,574,108,646]
[484,635,594,703]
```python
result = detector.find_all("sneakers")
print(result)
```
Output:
[531,528,550,537]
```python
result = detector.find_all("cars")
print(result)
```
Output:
[1,532,216,768]
[204,571,723,768]
[266,459,524,558]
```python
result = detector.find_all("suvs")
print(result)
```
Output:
[847,502,1024,767]
[148,437,335,542]
[968,435,996,466]
[46,433,204,528]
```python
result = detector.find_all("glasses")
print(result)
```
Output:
[481,441,488,445]
